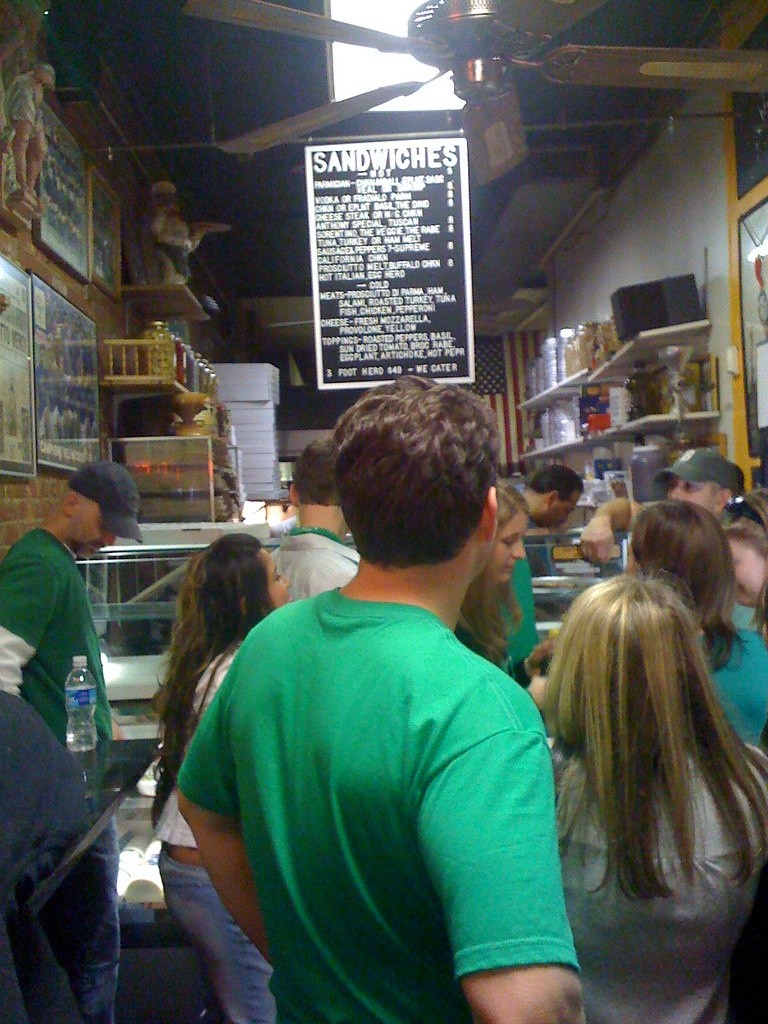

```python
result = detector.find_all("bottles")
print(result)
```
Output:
[526,320,720,506]
[141,321,220,397]
[64,656,97,752]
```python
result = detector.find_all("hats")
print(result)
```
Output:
[656,447,744,497]
[68,462,143,544]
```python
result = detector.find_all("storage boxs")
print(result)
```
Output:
[214,363,281,501]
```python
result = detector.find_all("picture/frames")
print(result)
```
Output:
[89,162,124,300]
[25,269,102,473]
[0,254,38,480]
[34,100,95,284]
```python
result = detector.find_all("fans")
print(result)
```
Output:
[180,0,767,168]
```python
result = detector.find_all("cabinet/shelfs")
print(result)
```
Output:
[516,321,716,510]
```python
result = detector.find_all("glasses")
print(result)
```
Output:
[723,495,764,525]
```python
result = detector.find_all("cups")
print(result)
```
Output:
[117,837,166,903]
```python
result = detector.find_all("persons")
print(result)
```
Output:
[145,534,290,1024]
[174,375,588,1024]
[535,563,768,1023]
[271,437,364,604]
[4,60,57,211]
[455,446,767,757]
[0,459,144,1024]
